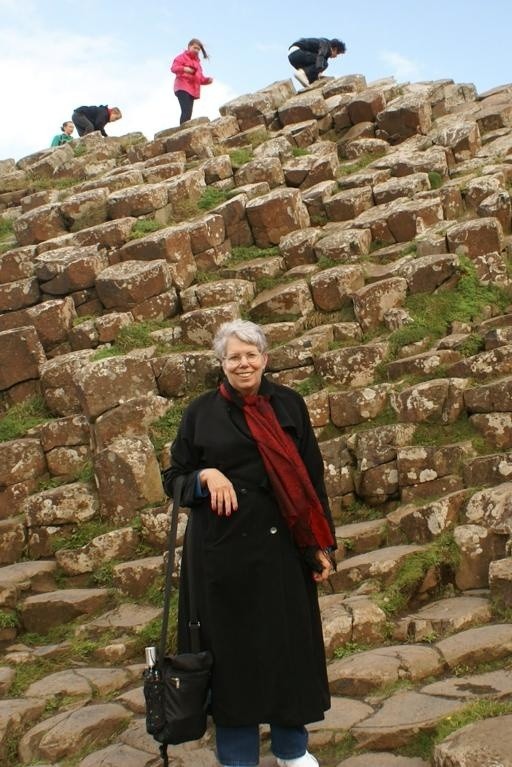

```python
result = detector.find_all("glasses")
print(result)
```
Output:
[222,351,264,364]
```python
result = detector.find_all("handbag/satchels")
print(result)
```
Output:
[140,643,216,746]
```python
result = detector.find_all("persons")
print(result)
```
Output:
[171,39,214,126]
[161,315,337,767]
[51,121,74,147]
[287,38,345,88]
[72,105,122,136]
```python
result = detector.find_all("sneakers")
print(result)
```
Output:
[273,750,321,767]
